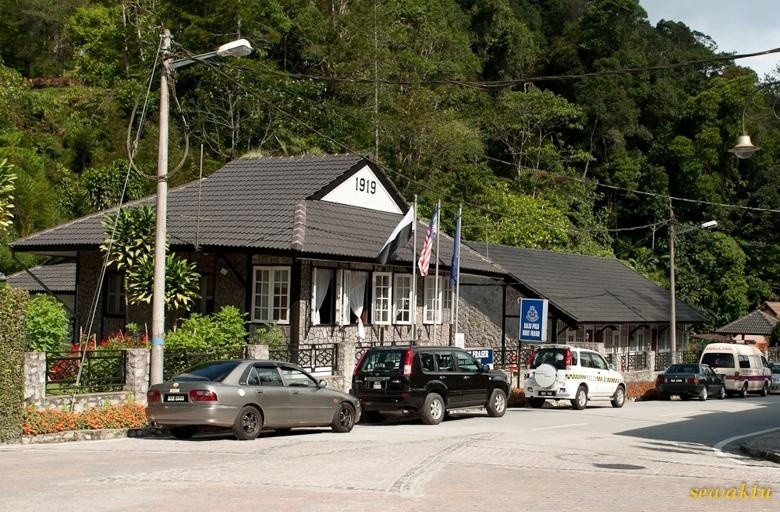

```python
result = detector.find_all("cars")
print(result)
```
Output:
[656,363,727,401]
[768,362,780,390]
[145,358,362,439]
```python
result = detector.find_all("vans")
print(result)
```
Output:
[698,342,773,397]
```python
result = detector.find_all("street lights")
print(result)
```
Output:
[149,29,254,385]
[669,220,718,363]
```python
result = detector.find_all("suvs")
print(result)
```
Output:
[349,344,511,424]
[523,342,627,410]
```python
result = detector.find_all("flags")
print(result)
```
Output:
[377,204,417,267]
[449,210,460,285]
[418,206,440,277]
